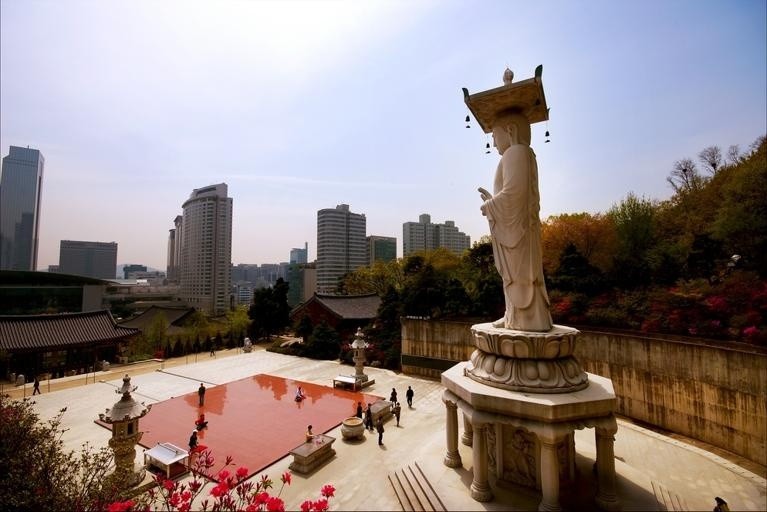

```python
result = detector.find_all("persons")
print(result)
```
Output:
[366,402,374,431]
[293,386,305,403]
[712,496,730,512]
[406,385,414,407]
[194,413,210,431]
[376,414,385,445]
[187,429,199,450]
[390,388,398,408]
[477,110,556,332]
[392,401,402,428]
[209,346,215,356]
[306,425,315,442]
[31,377,41,396]
[198,382,205,407]
[356,401,364,419]
